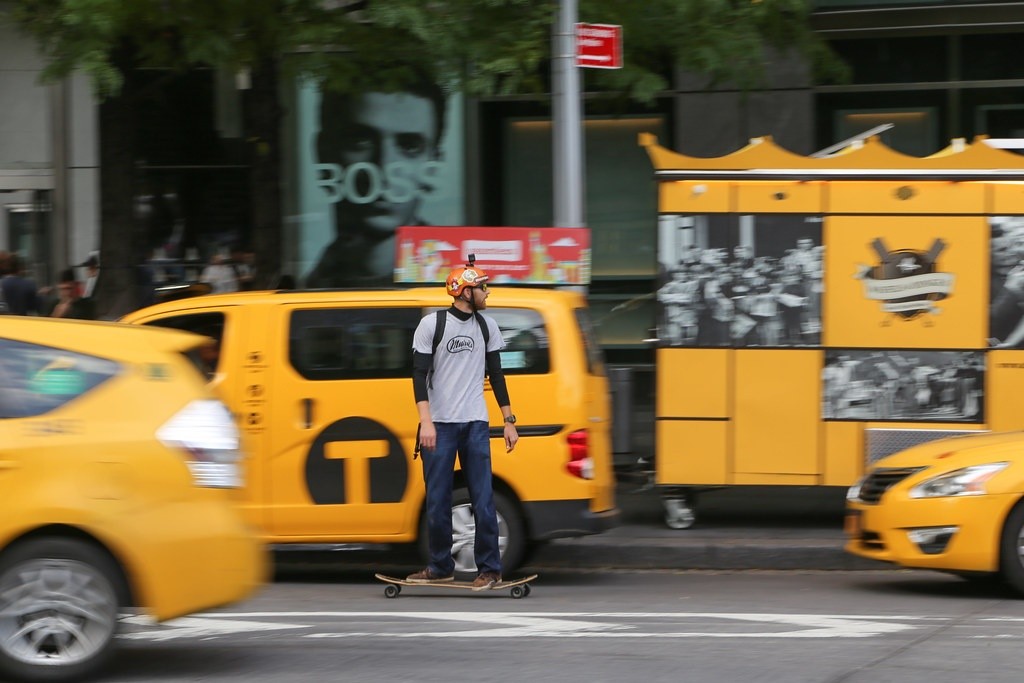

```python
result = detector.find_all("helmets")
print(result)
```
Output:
[445,253,489,296]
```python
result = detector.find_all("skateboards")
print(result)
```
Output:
[375,573,538,599]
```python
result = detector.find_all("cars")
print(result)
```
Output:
[0,312,276,683]
[841,429,1024,599]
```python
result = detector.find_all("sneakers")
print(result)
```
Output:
[472,570,502,592]
[405,568,455,583]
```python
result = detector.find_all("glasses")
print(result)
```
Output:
[470,283,487,292]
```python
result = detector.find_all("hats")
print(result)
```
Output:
[73,255,99,268]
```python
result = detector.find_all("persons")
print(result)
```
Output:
[0,239,279,377]
[406,266,519,590]
[657,222,1024,422]
[304,63,447,287]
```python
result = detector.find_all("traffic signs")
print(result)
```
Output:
[574,24,623,68]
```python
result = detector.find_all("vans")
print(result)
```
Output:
[33,281,621,581]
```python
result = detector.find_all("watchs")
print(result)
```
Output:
[504,415,517,423]
